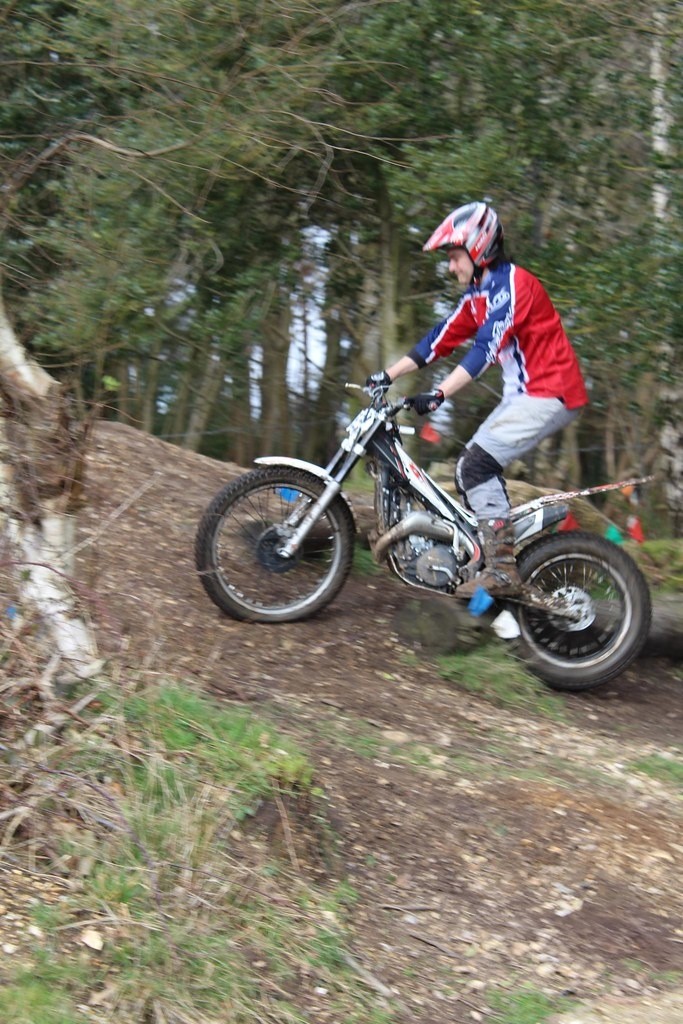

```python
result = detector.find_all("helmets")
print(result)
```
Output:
[423,202,504,283]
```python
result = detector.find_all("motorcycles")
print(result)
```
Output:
[195,380,659,690]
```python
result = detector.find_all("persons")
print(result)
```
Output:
[362,201,589,600]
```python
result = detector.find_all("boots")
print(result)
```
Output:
[454,516,522,597]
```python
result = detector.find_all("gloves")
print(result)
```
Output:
[403,388,443,415]
[366,370,392,393]
